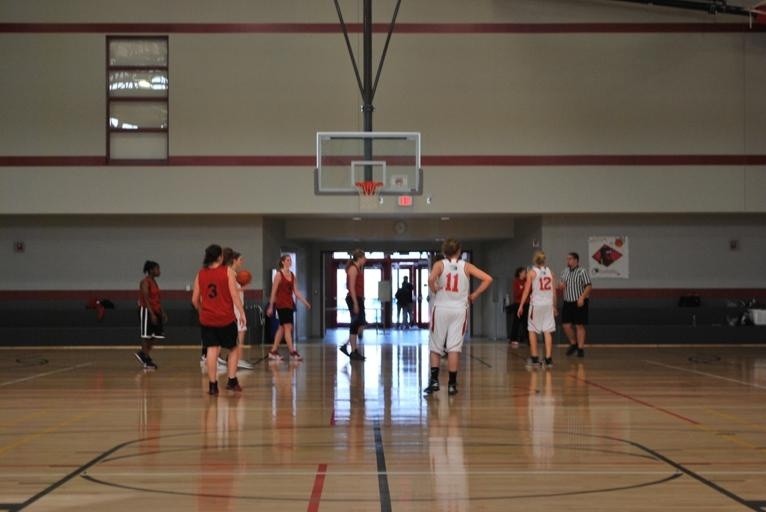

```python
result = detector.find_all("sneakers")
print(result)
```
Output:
[423,378,439,393]
[198,354,253,369]
[448,380,458,395]
[527,359,551,368]
[576,350,585,358]
[227,377,242,391]
[340,344,349,357]
[566,343,577,356]
[289,351,303,361]
[350,349,366,361]
[135,351,157,369]
[268,351,284,361]
[209,383,219,393]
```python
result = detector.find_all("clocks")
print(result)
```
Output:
[392,221,407,237]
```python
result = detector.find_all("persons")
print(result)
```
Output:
[427,254,448,357]
[517,248,559,368]
[192,243,254,395]
[135,261,165,369]
[266,255,311,361]
[395,276,418,329]
[341,250,366,360]
[559,253,593,357]
[510,267,531,345]
[425,239,493,394]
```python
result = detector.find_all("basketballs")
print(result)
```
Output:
[236,270,251,285]
